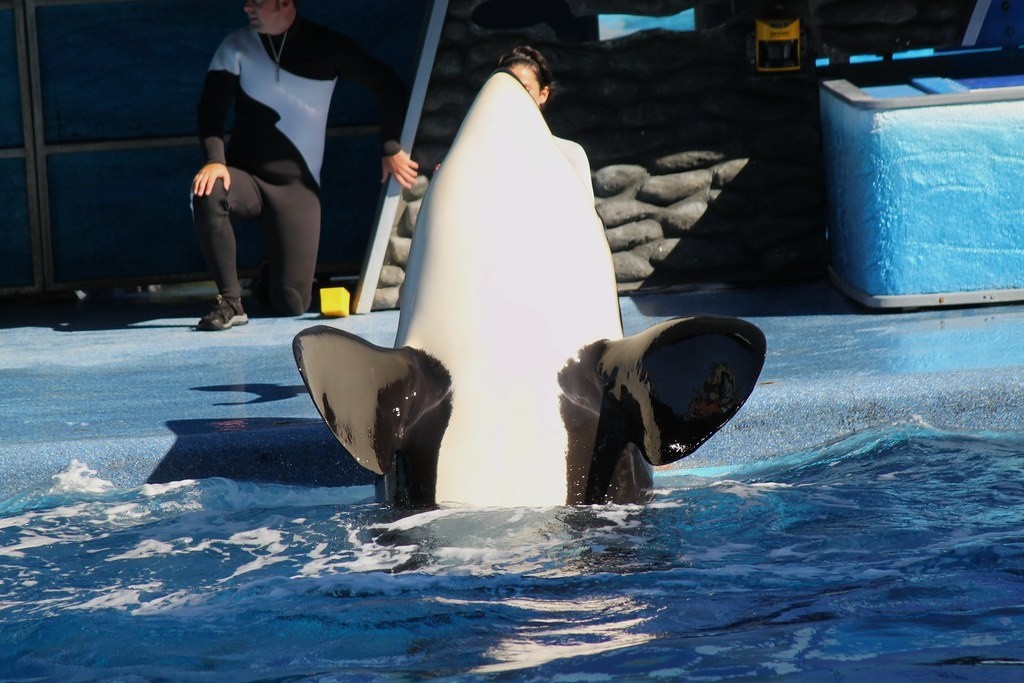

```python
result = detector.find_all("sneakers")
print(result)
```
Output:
[197,295,248,331]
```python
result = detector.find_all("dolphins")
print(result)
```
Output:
[290,70,771,516]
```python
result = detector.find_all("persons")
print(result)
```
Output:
[430,46,580,180]
[186,1,421,334]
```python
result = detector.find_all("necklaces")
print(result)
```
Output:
[262,33,290,84]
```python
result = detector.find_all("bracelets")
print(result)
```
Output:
[208,159,226,166]
